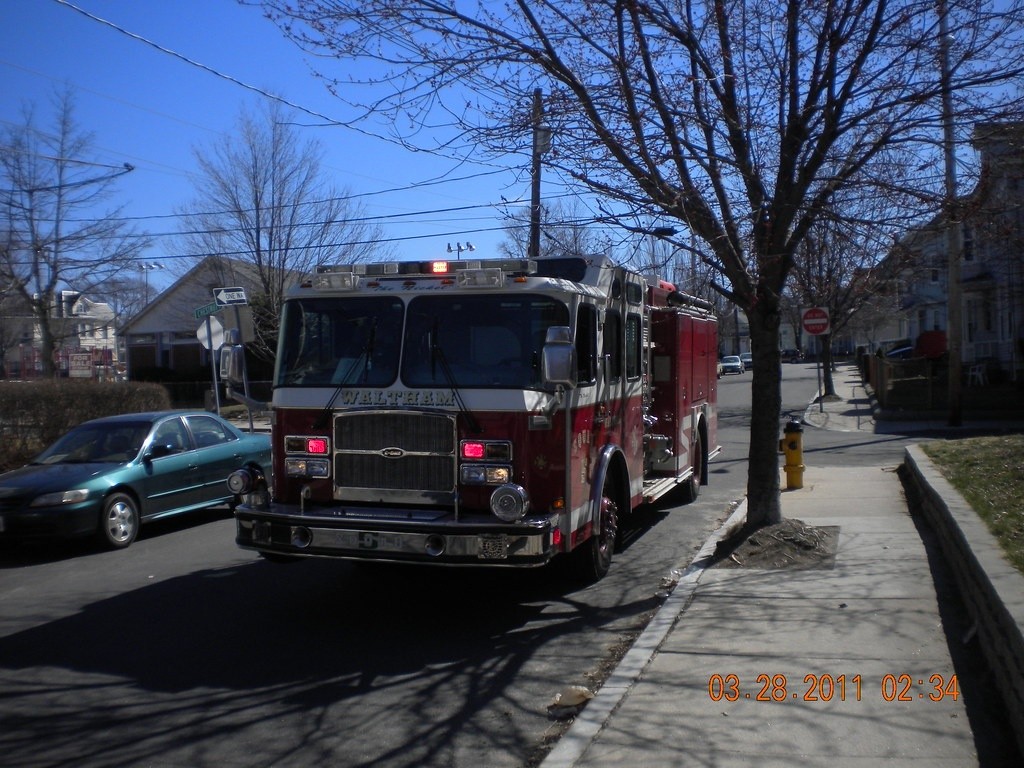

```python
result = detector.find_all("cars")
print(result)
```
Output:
[722,355,745,374]
[0,409,272,550]
[740,352,753,370]
[717,357,723,380]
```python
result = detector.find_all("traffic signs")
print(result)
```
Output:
[212,287,248,307]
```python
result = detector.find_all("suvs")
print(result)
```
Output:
[781,348,802,364]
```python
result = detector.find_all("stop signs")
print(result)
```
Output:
[801,307,831,336]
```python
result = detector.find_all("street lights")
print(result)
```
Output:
[446,241,476,260]
[137,260,165,307]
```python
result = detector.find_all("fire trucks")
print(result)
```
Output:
[226,252,723,595]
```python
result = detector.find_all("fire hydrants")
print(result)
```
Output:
[779,420,805,489]
